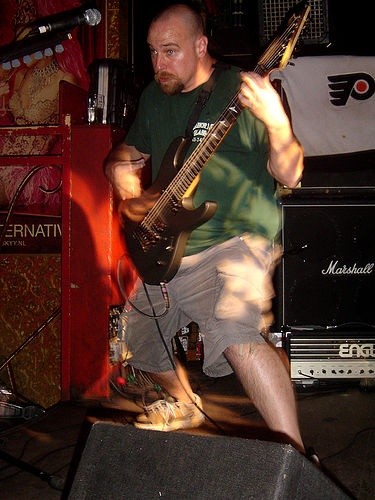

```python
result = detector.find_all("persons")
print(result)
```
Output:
[103,4,321,468]
[0,19,91,205]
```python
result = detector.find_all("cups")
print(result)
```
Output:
[88,94,104,124]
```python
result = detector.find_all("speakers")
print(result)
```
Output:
[68,421,353,500]
[276,202,375,336]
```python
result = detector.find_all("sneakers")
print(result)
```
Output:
[134,392,205,431]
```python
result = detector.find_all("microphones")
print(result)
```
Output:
[29,8,102,35]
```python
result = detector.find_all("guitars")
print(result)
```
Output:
[119,4,312,286]
